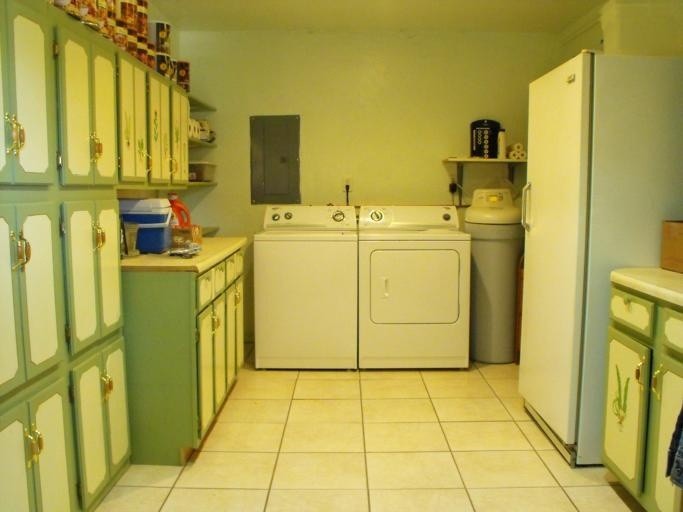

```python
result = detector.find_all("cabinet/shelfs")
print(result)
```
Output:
[60,197,126,360]
[170,81,189,186]
[71,335,132,509]
[55,23,117,186]
[1,199,59,399]
[146,73,173,186]
[114,52,146,184]
[122,233,246,465]
[0,376,79,510]
[0,1,55,189]
[602,265,682,512]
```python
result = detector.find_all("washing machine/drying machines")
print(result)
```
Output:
[253,204,358,370]
[359,204,472,369]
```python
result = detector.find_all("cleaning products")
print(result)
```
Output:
[167,192,191,228]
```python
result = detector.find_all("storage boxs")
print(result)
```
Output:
[117,198,174,254]
[190,161,216,182]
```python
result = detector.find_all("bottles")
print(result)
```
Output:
[198,122,210,142]
[497,129,507,160]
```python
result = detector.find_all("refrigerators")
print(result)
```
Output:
[518,47,682,469]
